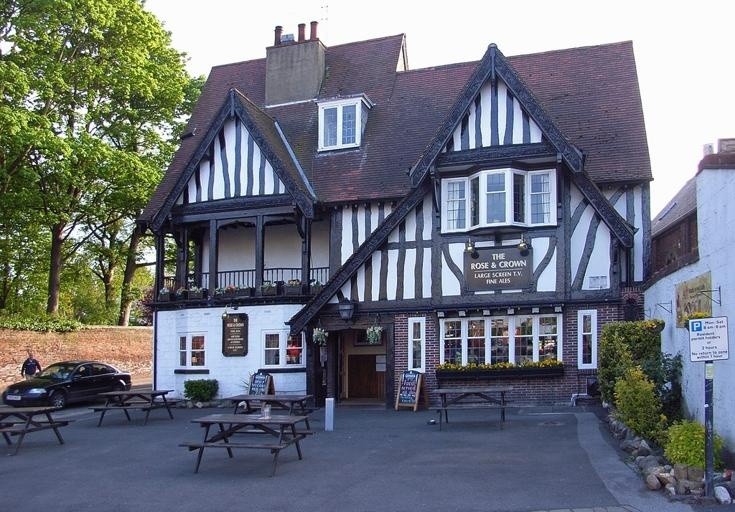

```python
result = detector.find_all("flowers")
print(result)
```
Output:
[366,326,385,344]
[312,328,328,346]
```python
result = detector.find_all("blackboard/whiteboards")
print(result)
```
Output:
[459,248,533,292]
[222,314,247,356]
[397,371,421,405]
[248,374,269,406]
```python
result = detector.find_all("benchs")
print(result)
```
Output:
[87,400,181,427]
[249,407,320,429]
[427,399,515,431]
[177,429,315,479]
[0,419,76,455]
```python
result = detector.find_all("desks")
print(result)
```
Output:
[431,387,514,431]
[190,413,310,478]
[225,394,314,430]
[97,391,174,426]
[1,406,63,455]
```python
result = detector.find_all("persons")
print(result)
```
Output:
[20,353,41,381]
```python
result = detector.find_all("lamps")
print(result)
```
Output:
[465,236,475,254]
[517,233,531,252]
[335,297,356,322]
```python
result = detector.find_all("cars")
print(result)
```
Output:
[3,360,131,407]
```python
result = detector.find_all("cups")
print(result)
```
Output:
[264,405,271,420]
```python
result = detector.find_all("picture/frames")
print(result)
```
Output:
[353,330,384,347]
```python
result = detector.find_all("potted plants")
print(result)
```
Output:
[214,280,324,298]
[159,286,208,301]
